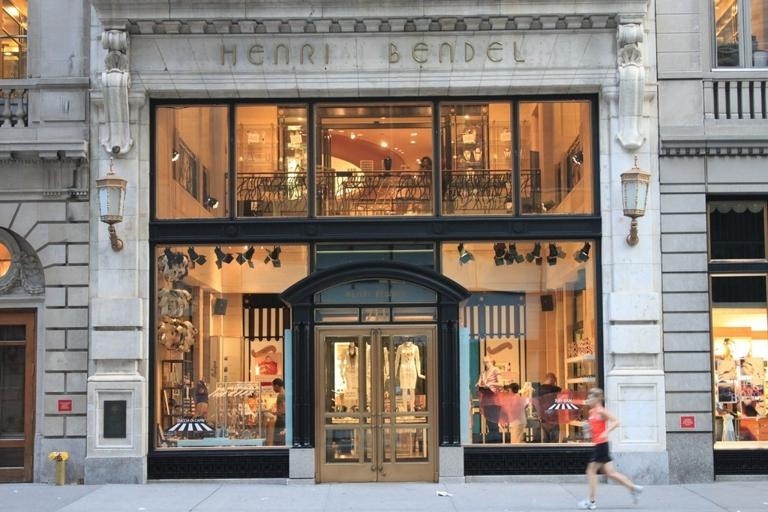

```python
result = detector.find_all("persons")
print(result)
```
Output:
[534,372,561,443]
[476,356,504,392]
[340,338,425,412]
[271,378,285,445]
[194,379,209,419]
[577,387,644,510]
[506,383,526,442]
[418,156,432,170]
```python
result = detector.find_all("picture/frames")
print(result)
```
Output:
[249,335,285,387]
[484,333,521,390]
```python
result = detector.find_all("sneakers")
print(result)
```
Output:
[630,485,644,505]
[578,500,596,511]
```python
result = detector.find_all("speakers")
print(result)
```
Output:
[214,298,227,315]
[540,294,553,311]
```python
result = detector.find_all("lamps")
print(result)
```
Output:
[620,155,652,246]
[163,243,283,270]
[455,238,592,266]
[95,155,128,252]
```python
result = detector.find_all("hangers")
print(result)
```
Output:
[207,381,257,399]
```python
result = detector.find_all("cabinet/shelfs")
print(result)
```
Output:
[564,353,598,441]
[162,359,192,430]
